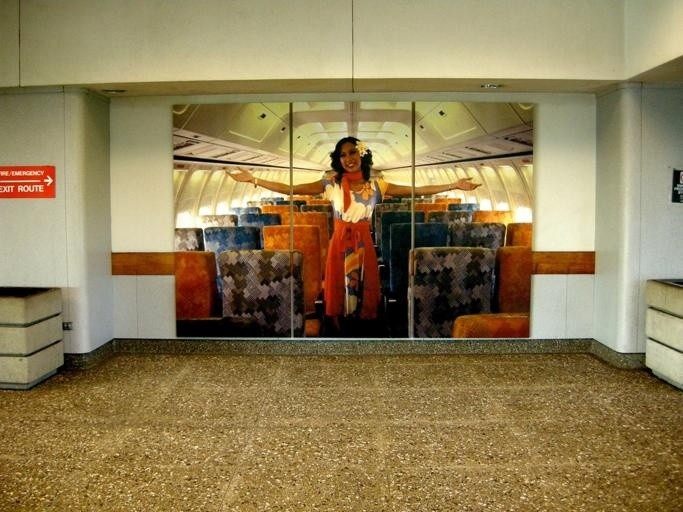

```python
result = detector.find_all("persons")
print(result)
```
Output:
[225,136,482,338]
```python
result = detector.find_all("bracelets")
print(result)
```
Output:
[254,177,258,188]
[448,184,451,191]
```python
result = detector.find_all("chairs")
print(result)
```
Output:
[174,193,335,339]
[372,194,532,338]
